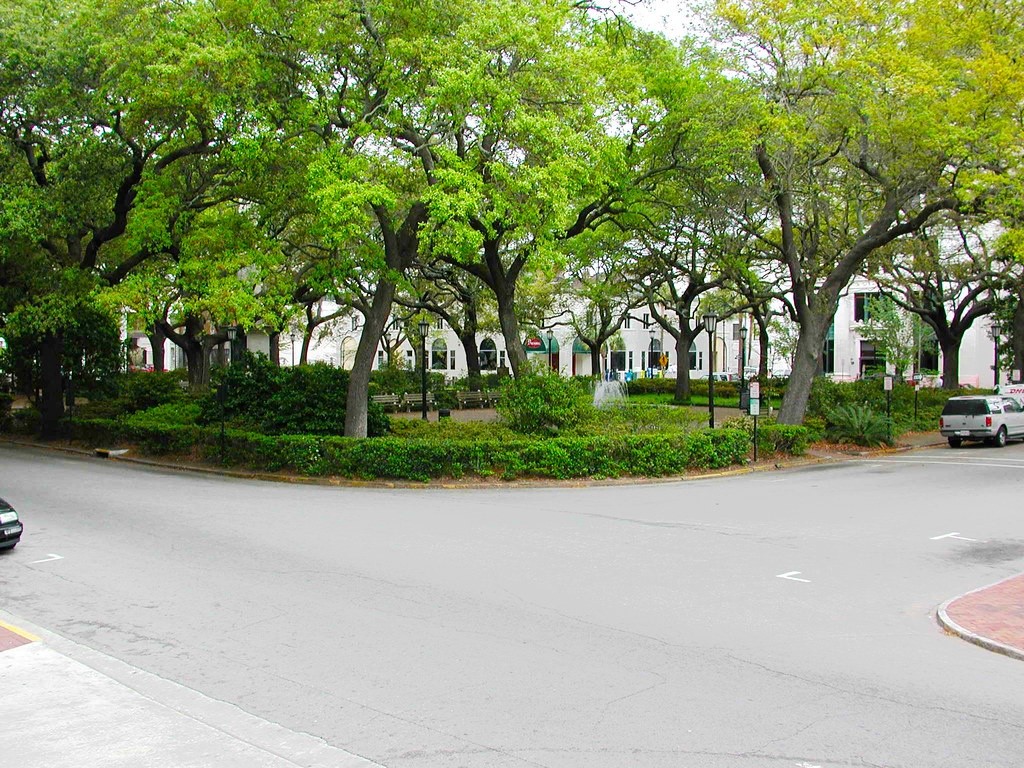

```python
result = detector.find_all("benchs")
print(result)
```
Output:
[403,391,435,412]
[456,389,487,409]
[487,390,504,408]
[371,392,401,414]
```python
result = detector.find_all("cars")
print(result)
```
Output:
[940,394,1024,447]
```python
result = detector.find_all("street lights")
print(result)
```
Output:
[991,318,1002,393]
[704,312,718,428]
[738,326,748,392]
[222,322,236,466]
[650,330,655,378]
[420,316,429,420]
[547,328,554,371]
